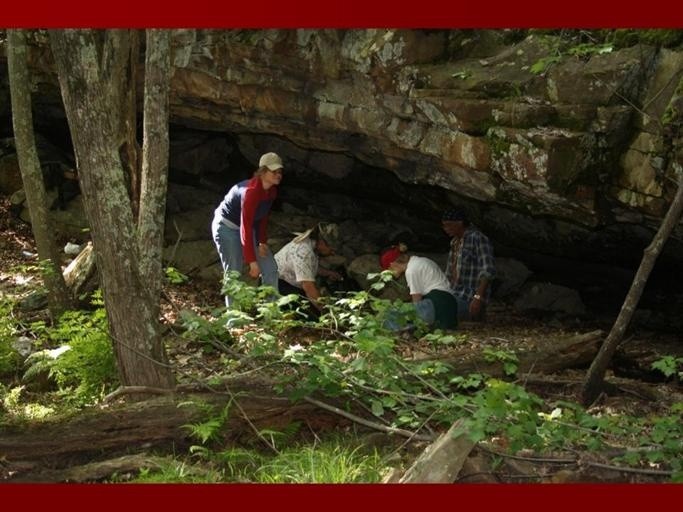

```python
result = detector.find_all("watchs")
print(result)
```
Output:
[472,292,484,303]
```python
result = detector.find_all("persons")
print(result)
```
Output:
[258,219,343,323]
[439,204,494,321]
[376,243,457,335]
[210,151,284,328]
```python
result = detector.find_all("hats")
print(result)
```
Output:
[259,152,284,170]
[380,247,401,270]
[319,221,342,251]
